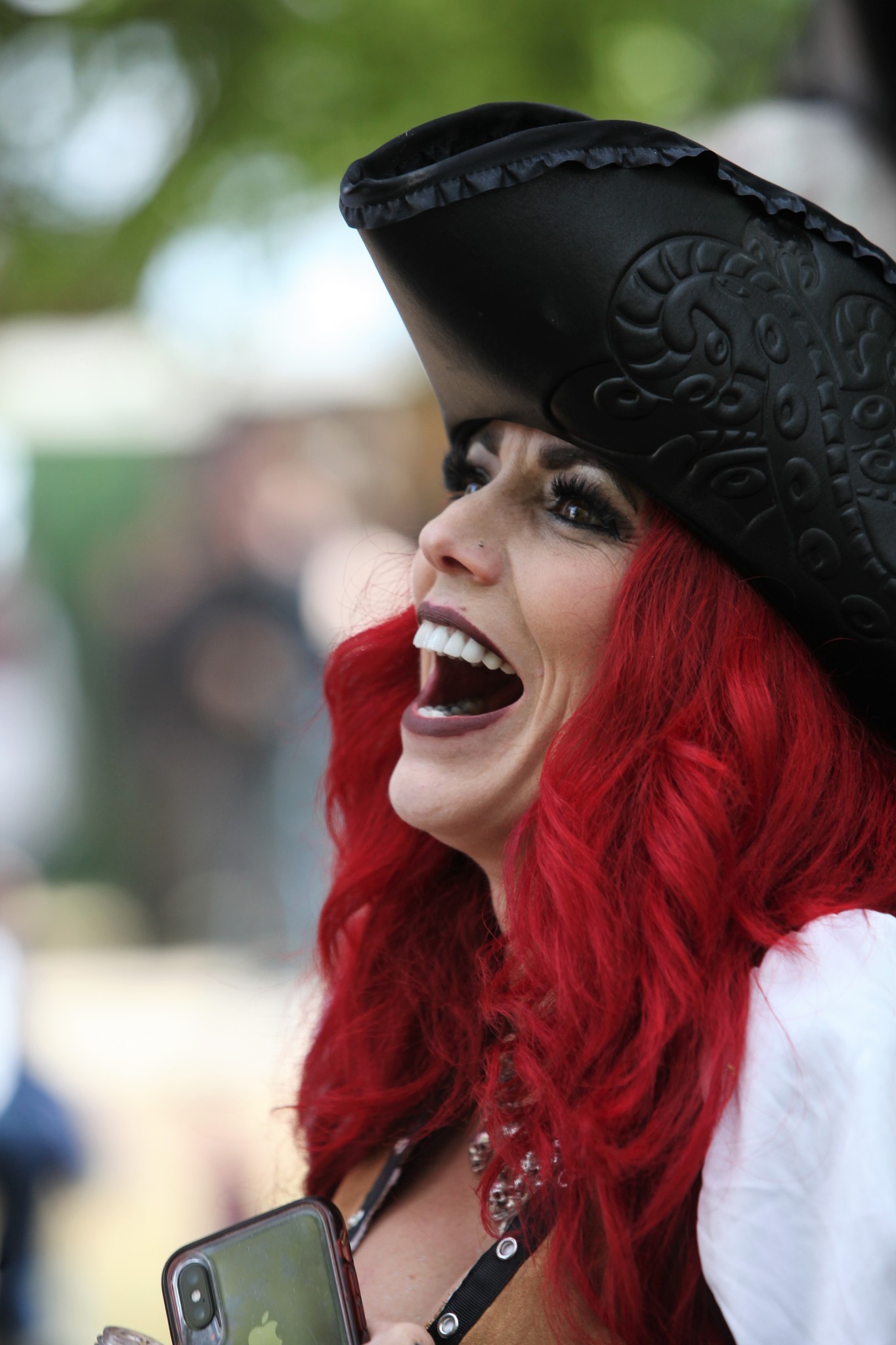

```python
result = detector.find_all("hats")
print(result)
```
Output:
[342,100,896,756]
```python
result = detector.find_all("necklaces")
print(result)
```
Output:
[466,1123,565,1226]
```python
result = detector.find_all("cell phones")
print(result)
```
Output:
[160,1195,369,1345]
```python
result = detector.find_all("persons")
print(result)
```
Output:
[272,102,896,1345]
